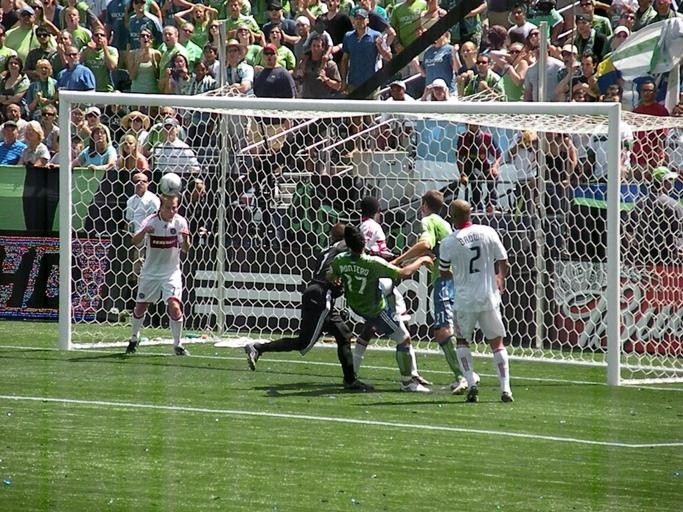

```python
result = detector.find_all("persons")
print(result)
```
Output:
[389,190,480,395]
[438,198,515,403]
[244,224,399,391]
[125,195,190,356]
[326,225,434,394]
[356,196,433,385]
[1,1,683,292]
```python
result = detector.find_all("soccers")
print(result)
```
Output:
[160,172,182,197]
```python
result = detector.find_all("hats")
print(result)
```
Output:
[561,44,578,54]
[389,81,407,92]
[429,79,446,87]
[650,167,679,183]
[121,111,151,131]
[266,0,280,8]
[162,118,177,127]
[353,8,368,18]
[85,107,101,115]
[225,39,248,58]
[263,44,277,53]
[614,26,630,35]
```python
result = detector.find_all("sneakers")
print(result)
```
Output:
[126,338,141,355]
[502,390,514,401]
[243,344,259,371]
[174,345,190,356]
[345,379,374,392]
[450,372,480,402]
[401,375,432,394]
